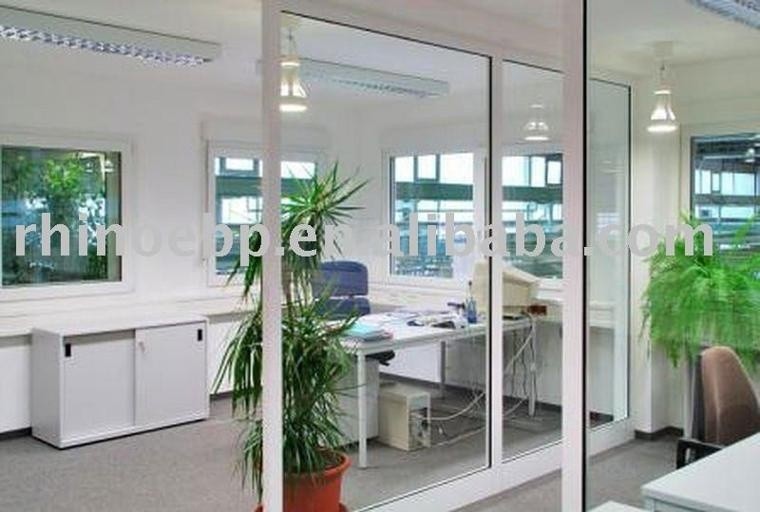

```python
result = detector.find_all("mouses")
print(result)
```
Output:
[407,320,424,326]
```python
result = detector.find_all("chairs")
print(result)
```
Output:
[675,344,760,470]
[310,259,399,386]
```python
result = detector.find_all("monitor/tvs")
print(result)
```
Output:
[471,259,539,319]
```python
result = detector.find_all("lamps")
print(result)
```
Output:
[645,63,680,136]
[521,102,553,144]
[279,14,310,114]
[745,142,757,165]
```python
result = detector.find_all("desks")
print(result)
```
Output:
[309,312,540,470]
[640,431,760,511]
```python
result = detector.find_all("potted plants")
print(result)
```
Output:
[209,158,369,512]
[638,214,760,463]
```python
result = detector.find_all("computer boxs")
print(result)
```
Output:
[373,383,431,451]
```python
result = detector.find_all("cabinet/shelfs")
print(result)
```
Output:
[30,313,211,451]
